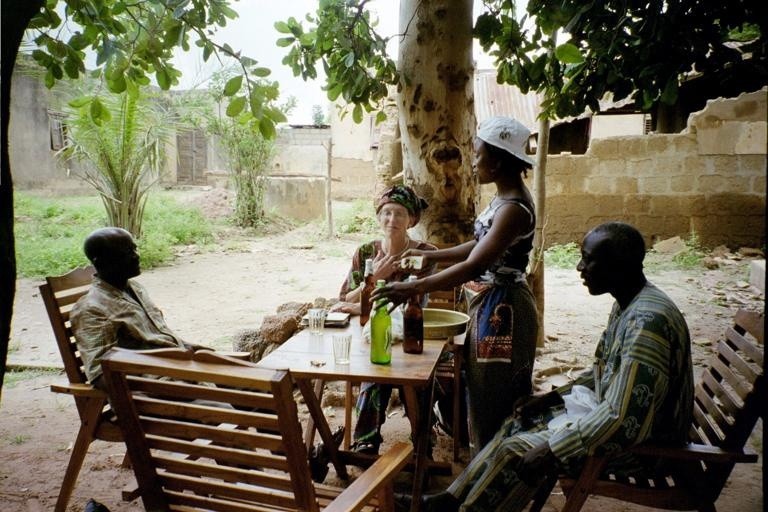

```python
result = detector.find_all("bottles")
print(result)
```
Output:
[360,259,375,326]
[402,275,424,354]
[369,280,392,365]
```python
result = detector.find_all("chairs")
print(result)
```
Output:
[38,264,255,512]
[100,347,415,512]
[343,240,463,463]
[529,309,766,512]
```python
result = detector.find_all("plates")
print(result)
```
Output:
[422,309,471,339]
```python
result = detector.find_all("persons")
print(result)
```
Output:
[330,184,439,450]
[69,226,345,483]
[394,223,695,512]
[370,116,539,453]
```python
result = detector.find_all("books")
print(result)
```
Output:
[300,310,351,329]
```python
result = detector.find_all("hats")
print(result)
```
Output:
[476,116,535,165]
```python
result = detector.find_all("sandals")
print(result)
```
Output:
[353,440,380,454]
[312,425,347,484]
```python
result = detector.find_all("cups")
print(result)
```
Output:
[308,309,326,335]
[332,332,352,366]
